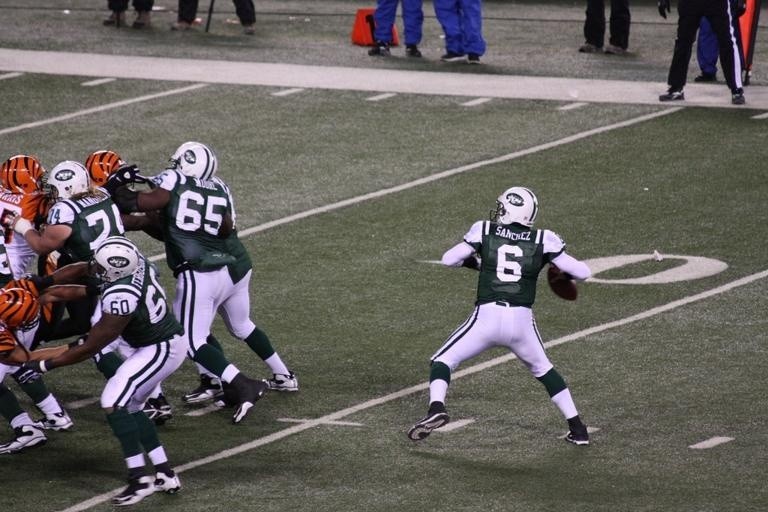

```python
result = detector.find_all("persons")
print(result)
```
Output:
[658,0,746,104]
[579,0,631,58]
[409,186,595,446]
[368,1,424,57]
[103,0,156,28]
[695,0,759,87]
[433,1,486,64]
[171,0,257,34]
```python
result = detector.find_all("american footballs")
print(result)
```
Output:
[547,268,577,300]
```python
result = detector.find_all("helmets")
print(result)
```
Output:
[0,155,47,195]
[89,234,140,281]
[146,141,218,189]
[495,186,540,228]
[46,160,91,198]
[84,149,125,188]
[0,278,41,328]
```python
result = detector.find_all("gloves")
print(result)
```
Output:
[117,163,145,184]
[658,0,671,19]
[731,0,746,18]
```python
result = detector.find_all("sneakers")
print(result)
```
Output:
[566,431,589,446]
[606,44,627,56]
[111,474,153,506]
[440,50,468,63]
[32,407,76,432]
[658,89,688,101]
[577,42,602,53]
[406,43,423,58]
[153,470,183,495]
[367,40,392,56]
[104,11,256,34]
[142,370,299,424]
[408,411,450,442]
[731,89,746,105]
[696,69,752,85]
[0,419,48,454]
[468,51,480,64]
